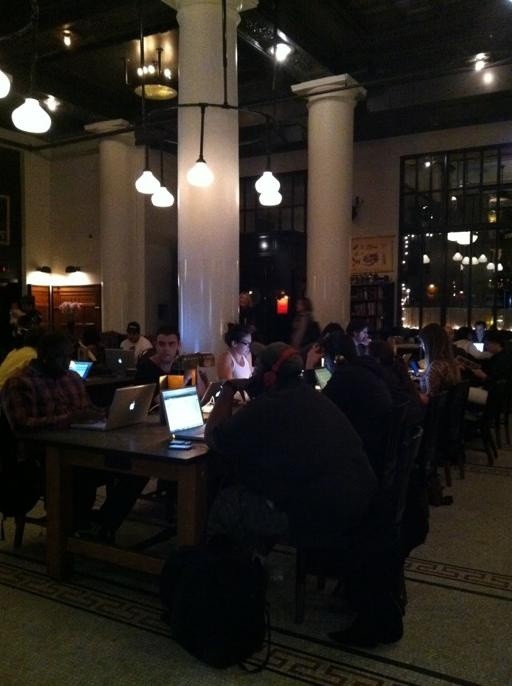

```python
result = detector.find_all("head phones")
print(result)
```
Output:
[329,335,350,371]
[255,347,300,393]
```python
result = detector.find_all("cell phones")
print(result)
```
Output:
[170,440,192,445]
[168,445,192,450]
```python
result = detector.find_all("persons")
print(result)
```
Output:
[215,321,256,382]
[203,342,380,570]
[283,297,321,344]
[119,321,155,368]
[301,330,395,477]
[1,332,152,547]
[240,289,262,337]
[0,295,100,387]
[384,322,510,508]
[133,325,192,415]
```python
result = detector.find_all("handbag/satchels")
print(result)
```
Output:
[155,548,270,669]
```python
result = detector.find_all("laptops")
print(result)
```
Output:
[70,382,157,431]
[105,350,135,368]
[200,381,223,406]
[409,360,425,371]
[472,342,485,353]
[314,367,334,391]
[68,360,94,381]
[161,386,207,441]
[402,353,412,366]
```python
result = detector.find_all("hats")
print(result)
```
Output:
[127,321,140,333]
[249,340,304,376]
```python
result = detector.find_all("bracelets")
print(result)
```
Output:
[222,380,236,391]
